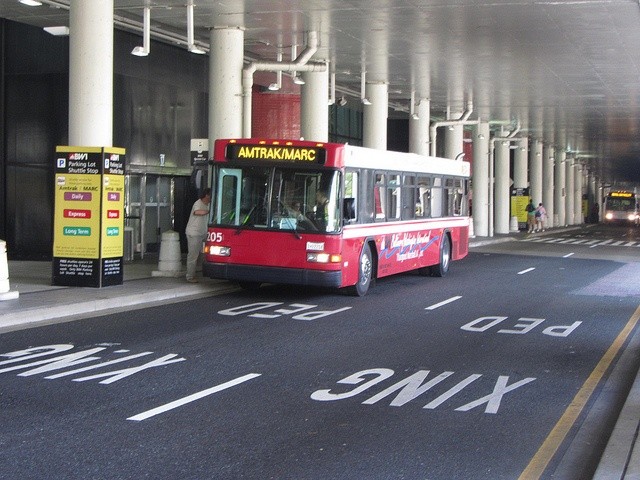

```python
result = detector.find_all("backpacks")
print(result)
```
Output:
[535,207,543,216]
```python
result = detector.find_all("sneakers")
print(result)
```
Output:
[186,278,198,284]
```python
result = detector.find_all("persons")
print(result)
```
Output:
[292,201,301,213]
[535,203,549,232]
[525,199,537,234]
[310,187,340,232]
[184,188,212,283]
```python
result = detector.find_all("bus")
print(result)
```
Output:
[202,139,470,297]
[603,192,640,224]
